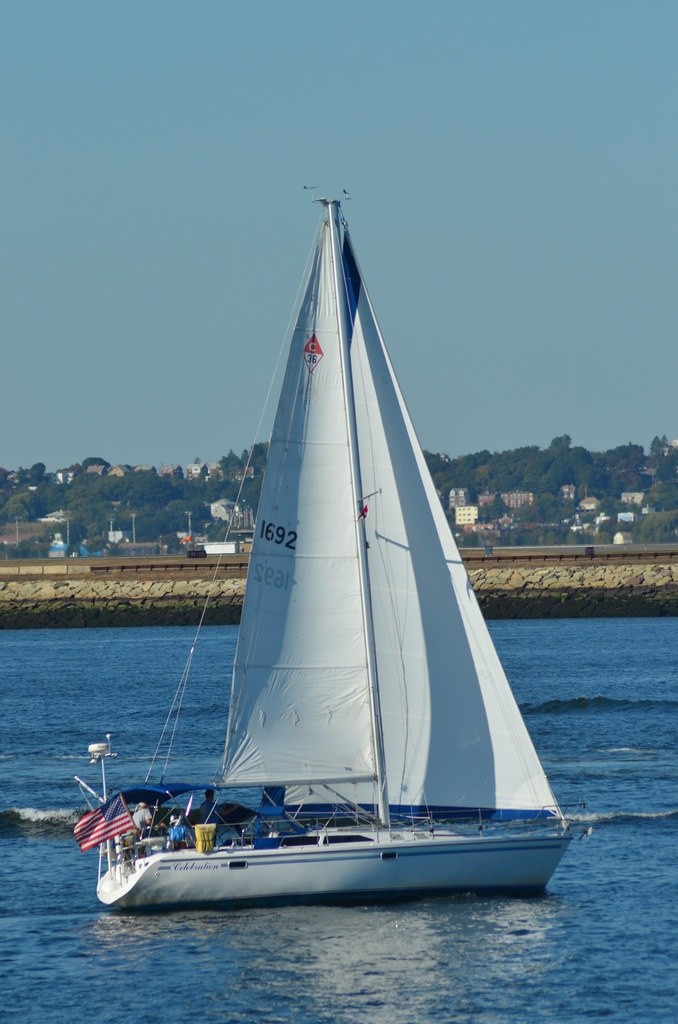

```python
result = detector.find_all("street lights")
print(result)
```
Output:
[185,512,192,536]
[14,517,20,545]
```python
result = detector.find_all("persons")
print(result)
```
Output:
[200,789,239,847]
[125,802,187,860]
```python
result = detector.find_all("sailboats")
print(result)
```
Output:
[72,197,588,908]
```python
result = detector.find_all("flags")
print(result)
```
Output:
[74,793,136,852]
